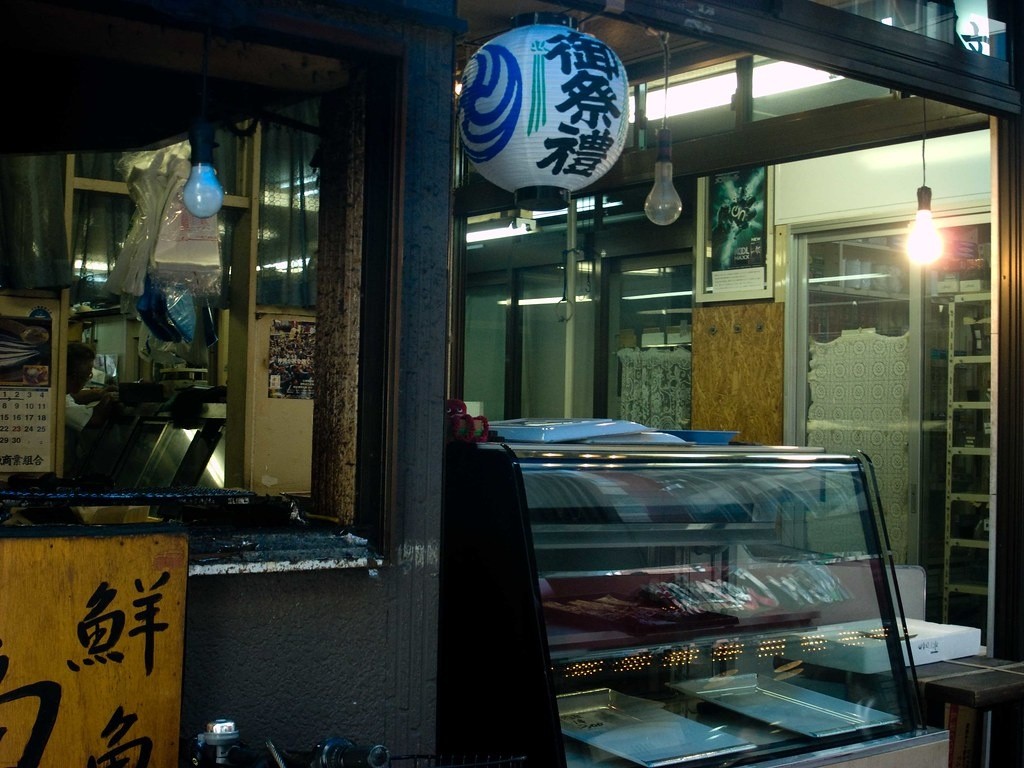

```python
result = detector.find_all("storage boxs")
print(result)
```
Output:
[619,335,637,347]
[936,282,959,293]
[667,331,691,343]
[642,332,665,345]
[960,279,989,293]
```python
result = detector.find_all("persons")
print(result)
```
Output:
[63,341,119,494]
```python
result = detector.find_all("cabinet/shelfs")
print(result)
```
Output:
[620,290,990,625]
[441,446,926,768]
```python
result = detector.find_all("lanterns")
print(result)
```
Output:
[459,10,631,210]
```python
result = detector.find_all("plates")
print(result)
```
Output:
[586,431,695,444]
[652,430,741,444]
[486,417,613,442]
